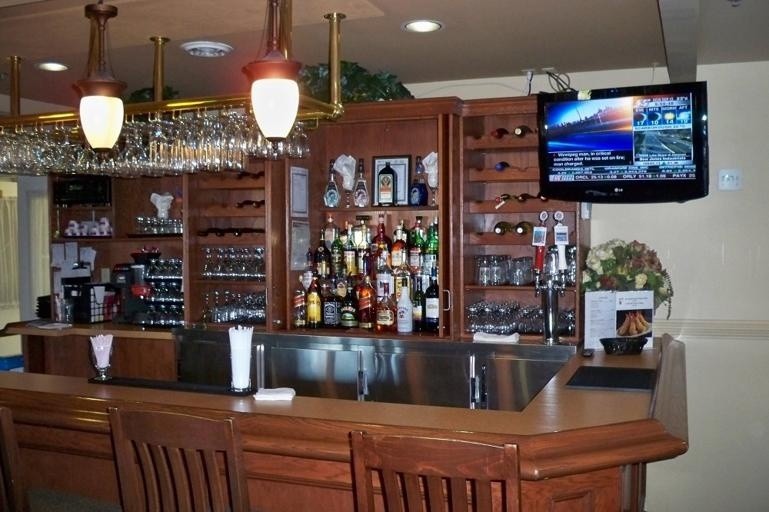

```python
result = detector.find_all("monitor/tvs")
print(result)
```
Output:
[537,80,708,206]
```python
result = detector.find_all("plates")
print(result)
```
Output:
[616,323,652,340]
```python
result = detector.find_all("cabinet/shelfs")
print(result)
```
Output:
[373,340,576,412]
[461,94,590,344]
[48,160,283,335]
[181,335,373,403]
[283,96,461,339]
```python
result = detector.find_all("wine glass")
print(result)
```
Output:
[463,299,575,337]
[90,346,115,381]
[143,246,266,332]
[0,102,313,177]
[133,217,183,234]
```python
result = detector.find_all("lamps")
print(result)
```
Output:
[241,0,303,144]
[71,0,129,153]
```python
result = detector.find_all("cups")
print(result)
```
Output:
[474,246,576,287]
[227,353,253,394]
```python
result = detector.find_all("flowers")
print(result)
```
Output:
[579,238,675,321]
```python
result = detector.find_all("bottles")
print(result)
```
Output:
[292,156,441,334]
[197,170,264,238]
[479,124,552,235]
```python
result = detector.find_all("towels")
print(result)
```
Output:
[253,387,296,404]
[472,331,520,345]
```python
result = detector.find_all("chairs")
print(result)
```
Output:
[350,429,522,511]
[0,406,32,512]
[109,401,251,512]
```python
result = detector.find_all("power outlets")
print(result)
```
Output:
[719,169,743,192]
